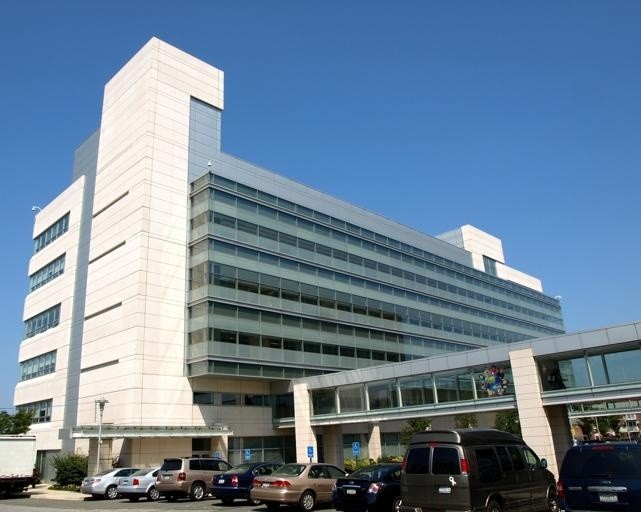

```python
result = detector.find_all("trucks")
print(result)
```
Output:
[1,435,40,494]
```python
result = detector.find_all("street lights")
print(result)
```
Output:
[94,394,111,476]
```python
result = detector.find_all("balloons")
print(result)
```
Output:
[478,364,509,397]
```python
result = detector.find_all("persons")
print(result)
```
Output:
[590,427,603,442]
